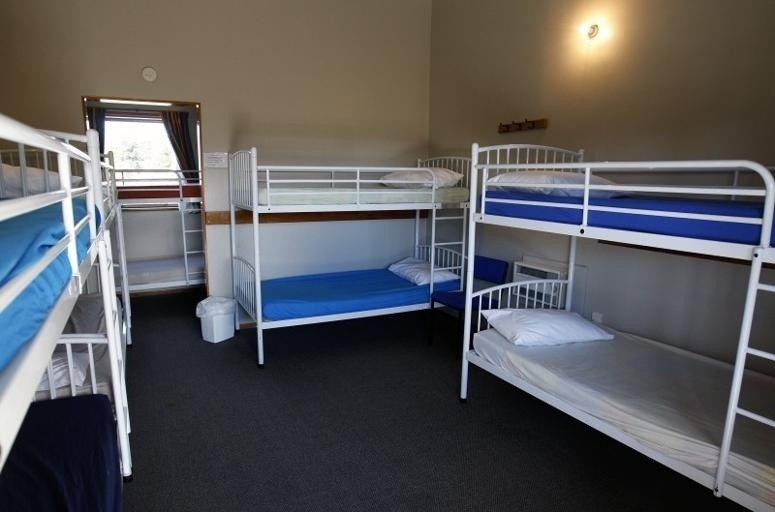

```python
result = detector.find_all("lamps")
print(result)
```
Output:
[574,20,603,45]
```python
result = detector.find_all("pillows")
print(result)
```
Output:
[485,170,636,200]
[386,257,461,287]
[478,307,615,347]
[379,167,464,189]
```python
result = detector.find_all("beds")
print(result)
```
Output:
[458,143,773,510]
[226,144,478,365]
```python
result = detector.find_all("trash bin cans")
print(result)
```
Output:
[200,296,240,343]
